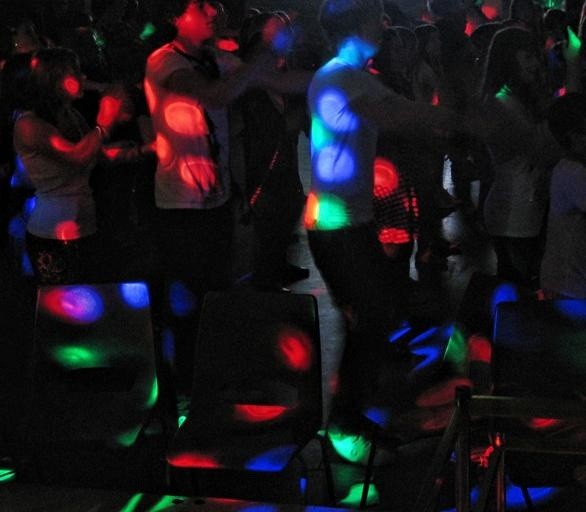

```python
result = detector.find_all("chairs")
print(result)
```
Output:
[24,281,162,450]
[361,286,584,509]
[162,287,338,506]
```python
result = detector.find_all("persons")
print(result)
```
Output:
[0,0,585,482]
